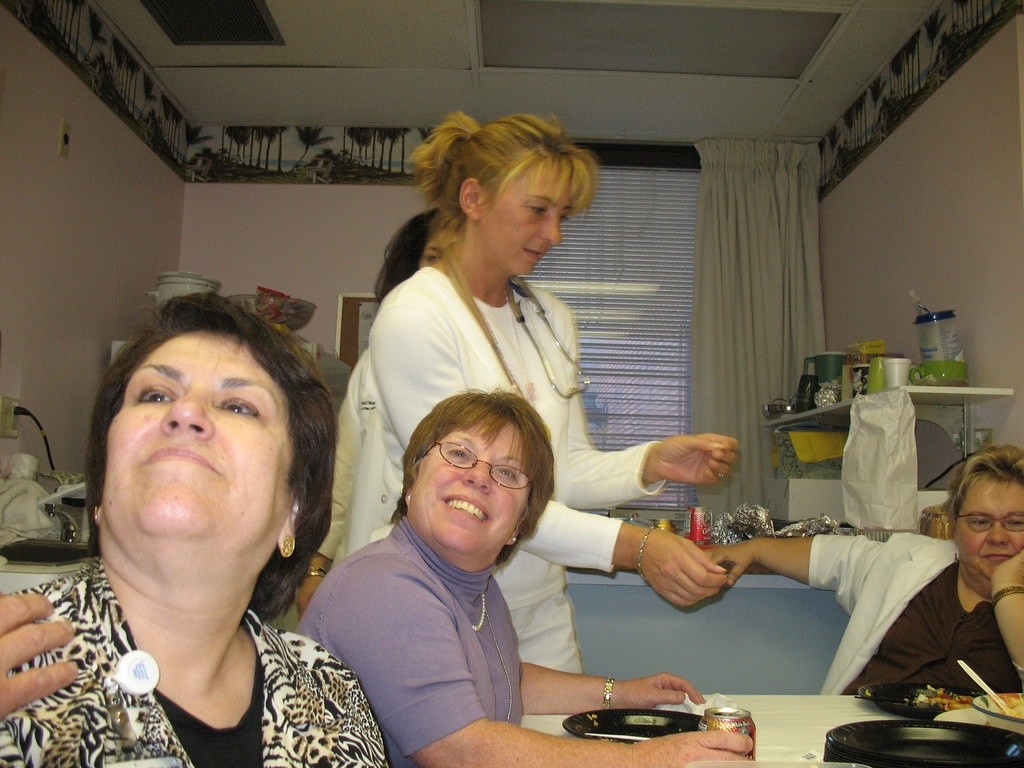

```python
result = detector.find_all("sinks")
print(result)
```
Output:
[0,540,98,567]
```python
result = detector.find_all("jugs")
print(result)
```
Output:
[803,351,854,383]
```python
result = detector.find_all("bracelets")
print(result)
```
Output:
[991,585,1024,608]
[603,677,615,710]
[637,528,660,582]
[306,565,327,576]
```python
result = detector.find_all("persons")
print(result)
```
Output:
[700,445,1024,697]
[0,289,389,768]
[297,385,753,768]
[297,207,461,617]
[347,108,744,675]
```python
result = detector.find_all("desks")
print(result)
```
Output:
[565,567,850,694]
[759,385,1014,522]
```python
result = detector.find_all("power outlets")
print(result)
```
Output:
[0,396,20,439]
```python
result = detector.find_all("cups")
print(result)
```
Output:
[880,358,911,387]
[912,309,965,361]
[12,452,40,479]
[796,375,818,413]
[867,357,891,395]
[909,360,968,386]
[852,364,869,398]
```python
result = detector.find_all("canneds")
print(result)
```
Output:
[796,374,818,412]
[683,506,711,547]
[651,518,676,534]
[698,707,756,760]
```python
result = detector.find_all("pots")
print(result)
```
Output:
[143,271,223,311]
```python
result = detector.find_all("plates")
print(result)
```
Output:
[562,709,703,745]
[933,707,985,725]
[860,683,978,720]
[823,720,1024,768]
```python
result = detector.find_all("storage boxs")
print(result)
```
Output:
[762,478,846,524]
[775,433,848,479]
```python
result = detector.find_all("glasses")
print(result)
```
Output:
[421,442,533,489]
[956,514,1024,532]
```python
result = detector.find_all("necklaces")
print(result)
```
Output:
[470,592,488,630]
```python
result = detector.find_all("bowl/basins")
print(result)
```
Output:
[971,693,1024,736]
[761,391,797,421]
[226,295,316,330]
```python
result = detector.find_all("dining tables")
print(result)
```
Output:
[520,694,913,768]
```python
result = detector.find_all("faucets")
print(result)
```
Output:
[44,503,81,541]
